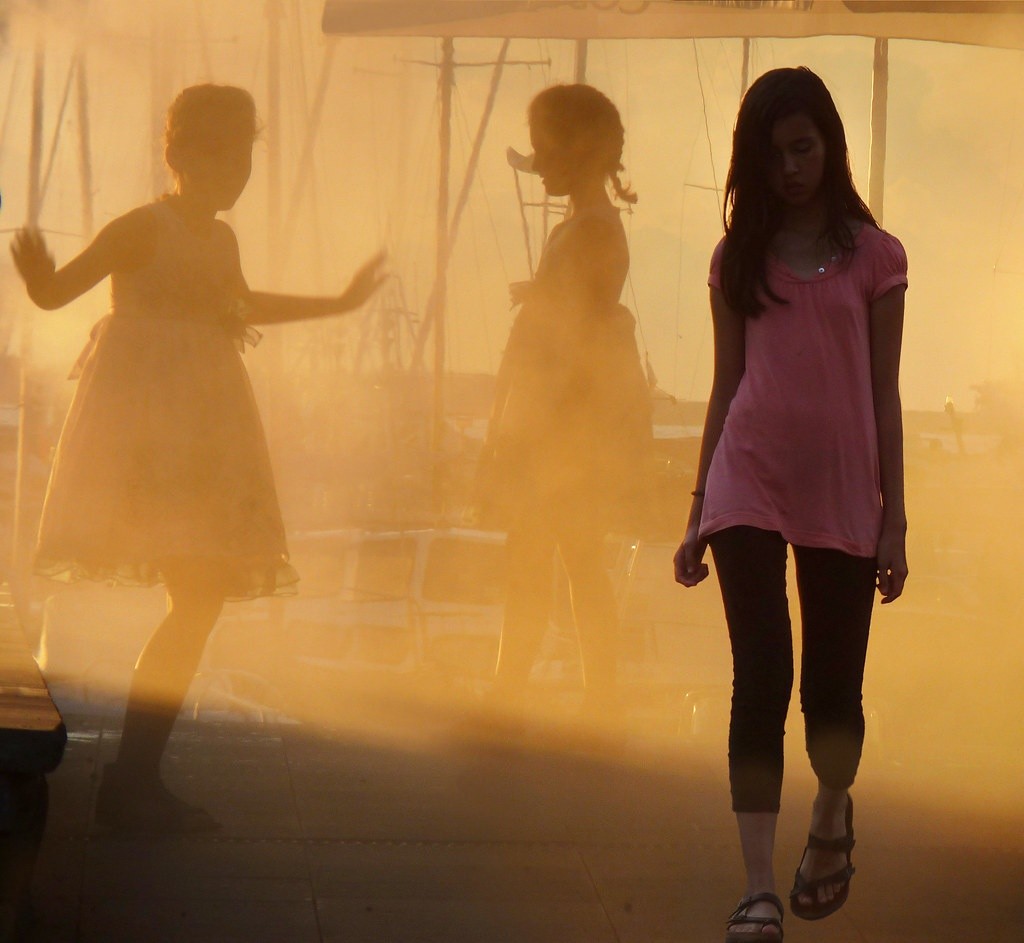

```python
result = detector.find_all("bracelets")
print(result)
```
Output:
[690,491,705,496]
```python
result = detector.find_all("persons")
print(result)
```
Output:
[670,67,908,942]
[466,83,654,734]
[7,83,392,838]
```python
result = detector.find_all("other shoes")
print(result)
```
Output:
[93,762,222,832]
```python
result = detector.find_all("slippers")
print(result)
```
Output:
[724,892,784,943]
[789,791,856,919]
